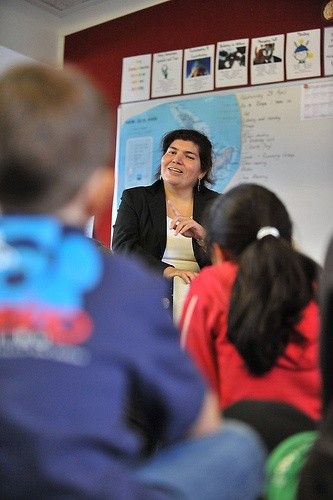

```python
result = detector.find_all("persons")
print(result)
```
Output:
[267,233,333,499]
[177,183,323,429]
[0,64,267,500]
[112,129,222,324]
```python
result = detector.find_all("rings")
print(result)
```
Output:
[173,220,180,230]
